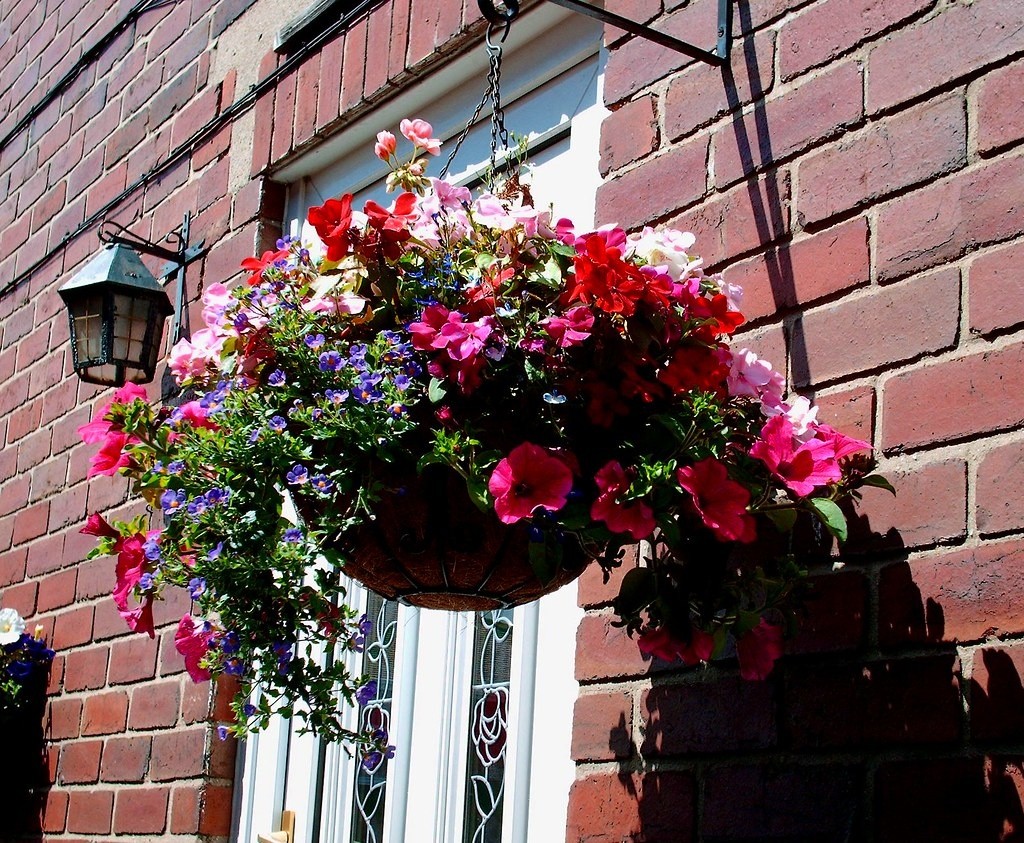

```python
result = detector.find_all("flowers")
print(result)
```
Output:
[71,117,875,768]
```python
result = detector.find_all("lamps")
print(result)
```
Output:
[56,211,208,389]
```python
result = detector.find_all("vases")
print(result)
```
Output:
[280,428,609,612]
